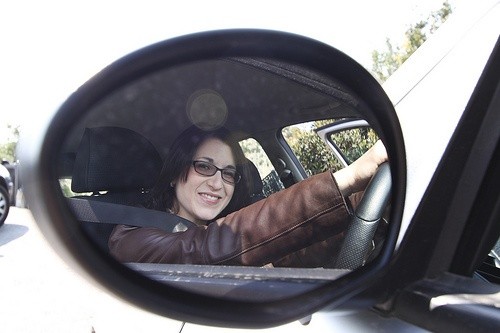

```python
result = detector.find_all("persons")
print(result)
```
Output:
[106,121,389,268]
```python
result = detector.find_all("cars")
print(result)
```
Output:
[0,162,14,228]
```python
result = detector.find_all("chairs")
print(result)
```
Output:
[240,155,266,208]
[68,125,156,259]
[127,146,176,211]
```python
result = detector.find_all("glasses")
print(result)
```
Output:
[191,160,241,185]
